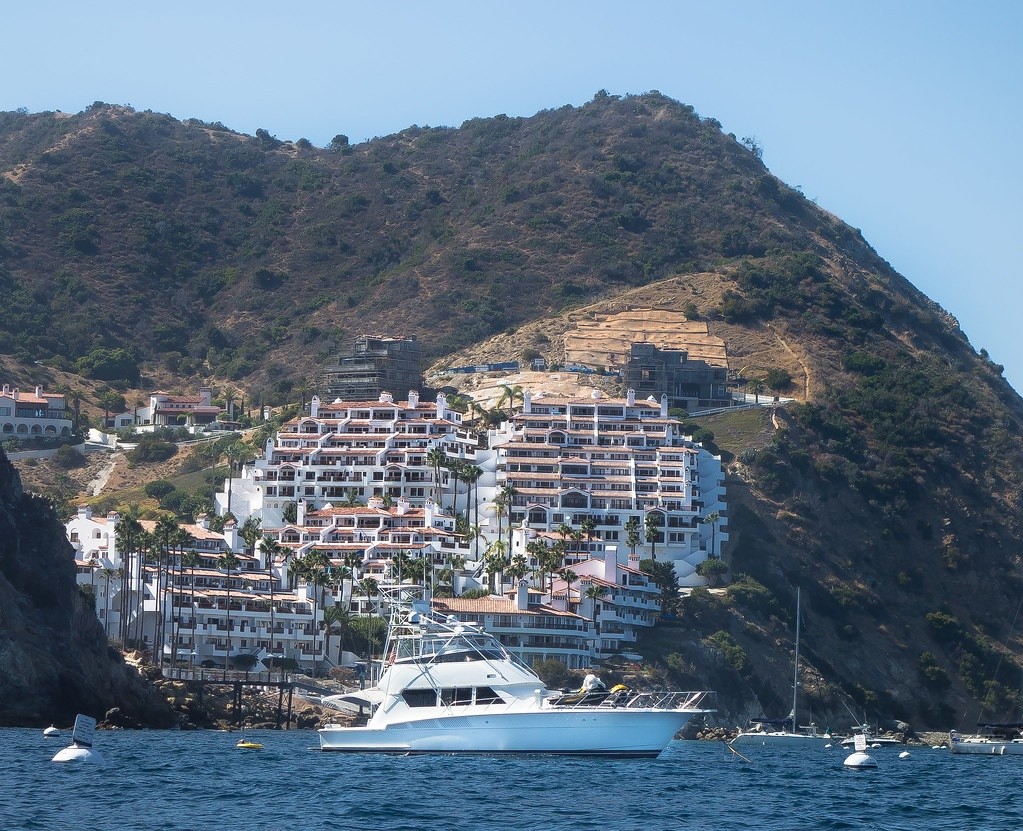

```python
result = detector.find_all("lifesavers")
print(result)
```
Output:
[236,742,264,748]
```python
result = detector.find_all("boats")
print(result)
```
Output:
[948,599,1023,755]
[837,696,902,747]
[237,740,264,749]
[548,680,613,709]
[317,509,719,759]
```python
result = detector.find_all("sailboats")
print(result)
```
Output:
[728,587,830,747]
